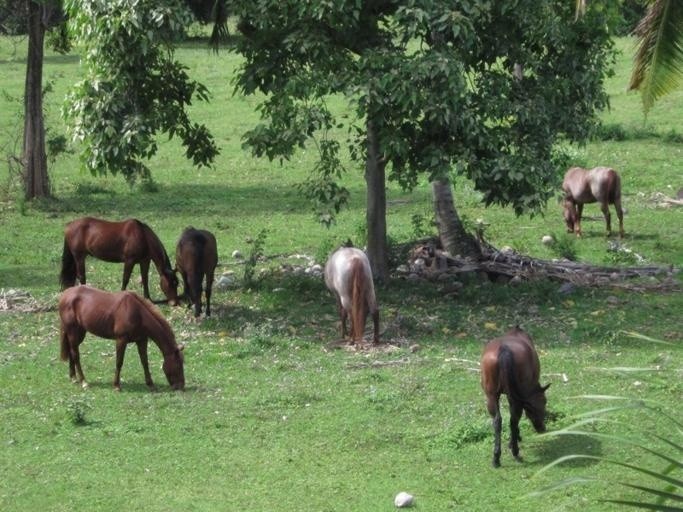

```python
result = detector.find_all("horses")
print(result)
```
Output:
[323,245,381,349]
[174,225,218,320]
[58,215,180,307]
[480,324,553,469]
[562,166,624,240]
[59,285,186,393]
